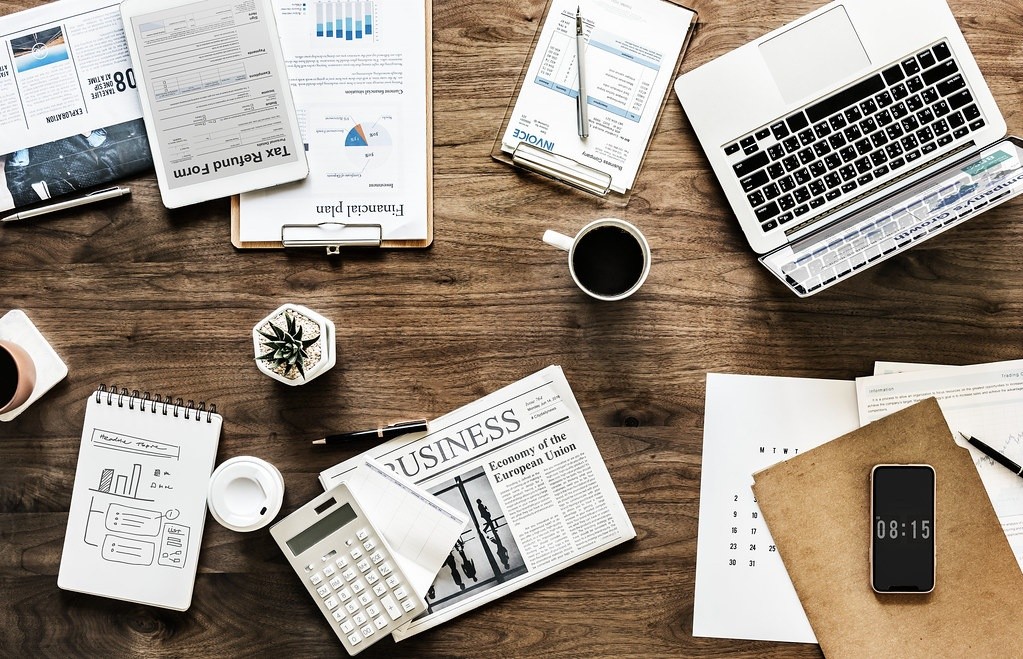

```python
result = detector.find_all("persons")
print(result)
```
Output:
[427,499,511,600]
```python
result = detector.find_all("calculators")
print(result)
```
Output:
[268,482,425,658]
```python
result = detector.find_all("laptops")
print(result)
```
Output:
[673,0,1023,299]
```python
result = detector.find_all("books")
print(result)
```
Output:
[54,378,224,612]
[753,395,1023,659]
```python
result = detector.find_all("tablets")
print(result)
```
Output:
[119,0,309,209]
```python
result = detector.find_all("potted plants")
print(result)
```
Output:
[252,303,337,385]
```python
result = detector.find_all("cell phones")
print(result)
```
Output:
[869,463,937,594]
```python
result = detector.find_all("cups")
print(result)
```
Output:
[542,217,652,301]
[208,455,286,534]
[0,338,37,414]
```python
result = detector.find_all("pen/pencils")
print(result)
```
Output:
[313,417,429,447]
[958,430,1023,481]
